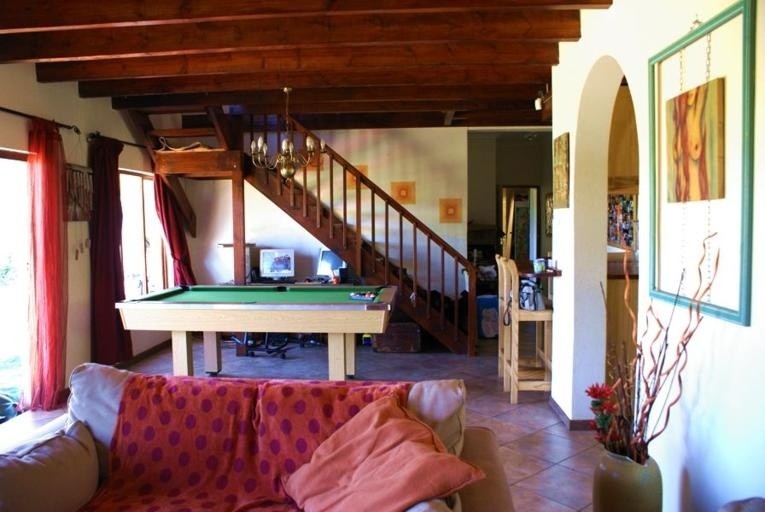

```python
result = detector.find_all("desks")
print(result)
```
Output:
[112,281,401,380]
[513,256,561,281]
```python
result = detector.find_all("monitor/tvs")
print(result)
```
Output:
[259,248,295,277]
[317,248,347,275]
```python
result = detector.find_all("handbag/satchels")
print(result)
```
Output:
[520,276,546,311]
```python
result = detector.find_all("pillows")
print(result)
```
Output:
[281,388,488,512]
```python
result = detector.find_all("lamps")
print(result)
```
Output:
[251,81,327,185]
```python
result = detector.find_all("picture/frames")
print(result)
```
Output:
[646,0,755,327]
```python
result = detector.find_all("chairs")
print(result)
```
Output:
[501,258,553,402]
[493,252,552,393]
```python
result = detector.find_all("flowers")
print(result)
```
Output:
[585,377,667,460]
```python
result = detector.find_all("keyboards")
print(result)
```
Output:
[262,279,296,283]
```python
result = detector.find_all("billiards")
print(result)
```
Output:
[350,291,377,298]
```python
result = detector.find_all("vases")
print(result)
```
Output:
[593,451,666,511]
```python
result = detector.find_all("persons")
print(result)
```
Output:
[671,84,711,202]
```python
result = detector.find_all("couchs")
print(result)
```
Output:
[2,361,521,510]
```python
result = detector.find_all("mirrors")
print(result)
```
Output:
[497,184,542,265]
